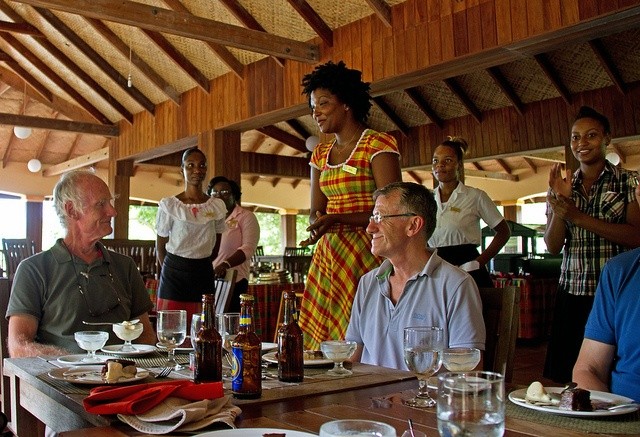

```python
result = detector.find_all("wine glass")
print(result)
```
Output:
[74,330,110,353]
[222,312,239,354]
[156,310,185,370]
[191,312,226,356]
[440,346,482,388]
[321,341,357,377]
[403,328,442,409]
[111,322,144,351]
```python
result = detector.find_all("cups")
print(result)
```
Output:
[317,419,397,435]
[437,370,504,436]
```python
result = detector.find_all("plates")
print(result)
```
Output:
[261,341,279,350]
[58,354,115,365]
[151,334,193,352]
[46,364,149,384]
[262,351,339,366]
[509,386,636,418]
[102,343,156,358]
[194,428,314,436]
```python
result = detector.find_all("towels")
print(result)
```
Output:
[82,378,225,417]
[116,393,243,435]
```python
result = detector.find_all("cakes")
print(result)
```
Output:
[101,357,136,383]
[558,388,590,411]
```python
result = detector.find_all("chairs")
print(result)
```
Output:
[273,290,304,343]
[468,284,520,384]
[286,247,307,284]
[247,246,266,271]
[4,239,40,295]
[106,239,159,274]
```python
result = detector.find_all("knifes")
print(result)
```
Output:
[594,403,637,411]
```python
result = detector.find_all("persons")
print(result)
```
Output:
[207,176,260,314]
[344,182,486,394]
[572,247,640,404]
[543,106,640,384]
[5,169,156,358]
[427,135,511,287]
[156,148,228,336]
[297,60,402,353]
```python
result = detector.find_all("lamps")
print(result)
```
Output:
[605,140,620,166]
[128,25,132,87]
[14,84,31,139]
[28,129,42,174]
[306,113,322,152]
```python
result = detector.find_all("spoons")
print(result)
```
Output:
[81,318,141,325]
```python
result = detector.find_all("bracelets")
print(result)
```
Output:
[223,260,231,267]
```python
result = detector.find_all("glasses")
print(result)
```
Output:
[368,210,419,224]
[61,238,123,319]
[209,189,231,198]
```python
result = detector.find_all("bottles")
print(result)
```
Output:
[277,290,305,382]
[231,294,263,398]
[194,293,223,382]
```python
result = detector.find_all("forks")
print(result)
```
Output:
[143,366,173,378]
[549,382,578,397]
